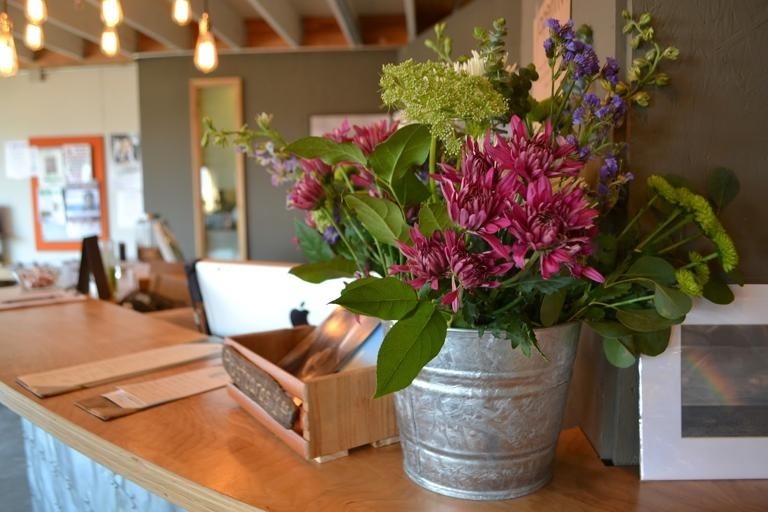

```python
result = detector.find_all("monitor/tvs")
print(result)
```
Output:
[185,257,358,339]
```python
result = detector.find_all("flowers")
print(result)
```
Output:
[197,1,737,399]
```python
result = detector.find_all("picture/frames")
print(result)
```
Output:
[638,283,768,481]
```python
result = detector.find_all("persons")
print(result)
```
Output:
[114,135,140,166]
[81,190,98,212]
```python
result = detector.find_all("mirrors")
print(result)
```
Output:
[188,77,248,261]
